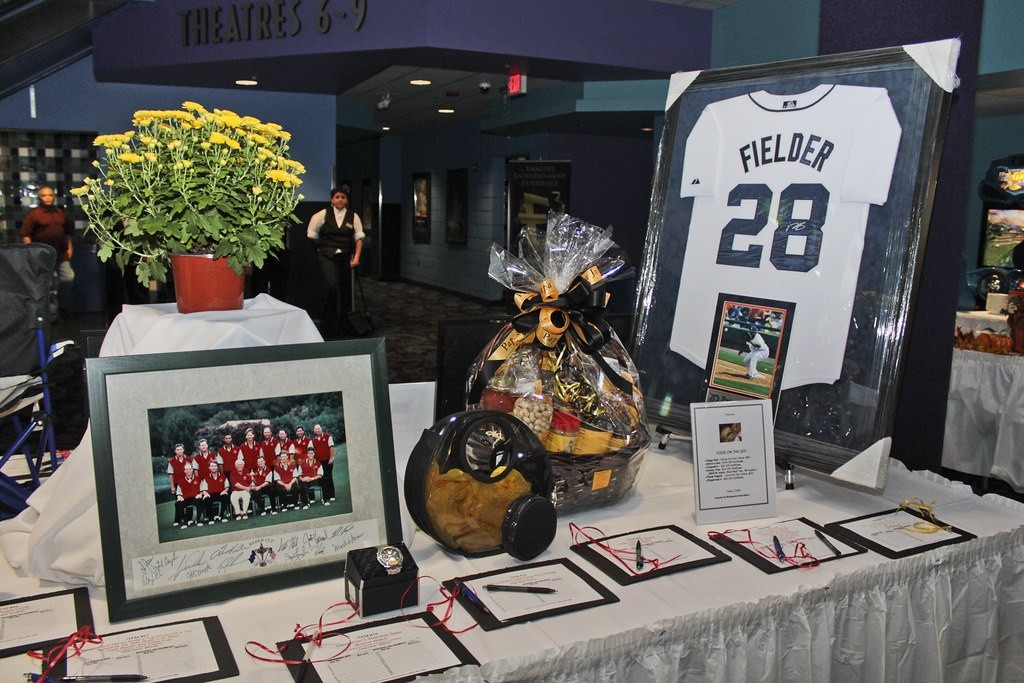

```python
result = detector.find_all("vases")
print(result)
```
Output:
[172,248,247,313]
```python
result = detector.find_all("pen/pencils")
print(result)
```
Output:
[482,584,559,594]
[814,530,841,556]
[60,675,150,683]
[773,536,786,563]
[636,540,643,570]
[24,672,57,683]
[453,577,489,614]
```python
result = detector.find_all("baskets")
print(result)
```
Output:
[466,322,652,517]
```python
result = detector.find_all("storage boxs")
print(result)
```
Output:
[343,543,420,611]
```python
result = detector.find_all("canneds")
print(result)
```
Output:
[542,411,580,456]
[483,375,519,415]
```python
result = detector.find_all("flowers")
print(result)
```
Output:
[72,103,318,264]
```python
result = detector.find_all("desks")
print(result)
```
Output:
[0,384,1024,683]
[941,311,1024,496]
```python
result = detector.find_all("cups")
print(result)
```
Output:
[985,293,1009,315]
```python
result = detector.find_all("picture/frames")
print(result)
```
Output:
[80,338,405,624]
[444,167,468,244]
[625,39,963,488]
[411,172,431,245]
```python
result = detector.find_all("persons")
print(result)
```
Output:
[20,186,74,323]
[306,188,366,303]
[167,424,335,529]
[725,306,782,379]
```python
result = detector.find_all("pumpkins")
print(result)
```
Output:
[977,333,1013,351]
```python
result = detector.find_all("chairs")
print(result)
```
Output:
[0,243,75,515]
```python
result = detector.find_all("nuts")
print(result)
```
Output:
[512,398,554,445]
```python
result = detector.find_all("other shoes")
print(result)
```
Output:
[173,497,335,530]
